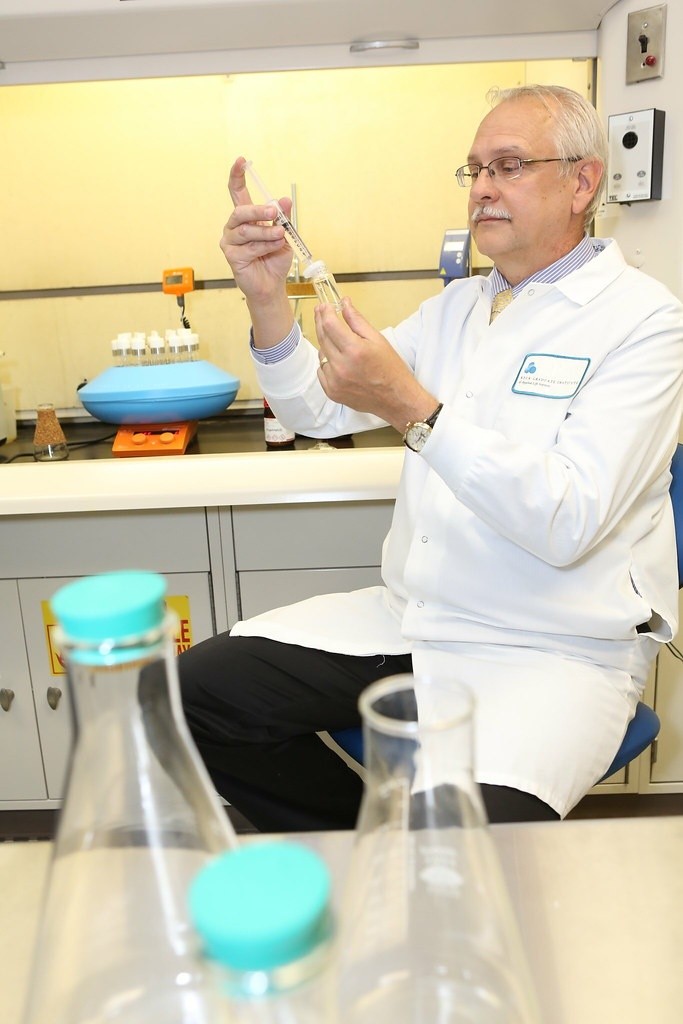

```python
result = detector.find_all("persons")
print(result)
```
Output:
[177,82,683,831]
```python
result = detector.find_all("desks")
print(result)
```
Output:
[0,815,682,1023]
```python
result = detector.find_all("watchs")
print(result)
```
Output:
[400,402,443,452]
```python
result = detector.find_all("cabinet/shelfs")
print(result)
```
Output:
[0,500,683,808]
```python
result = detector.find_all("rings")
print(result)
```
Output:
[320,357,327,369]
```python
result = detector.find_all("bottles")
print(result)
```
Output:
[111,330,201,367]
[34,403,69,461]
[25,568,239,1024]
[263,396,296,449]
[338,678,542,1024]
[185,837,351,1024]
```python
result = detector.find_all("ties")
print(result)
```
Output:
[489,289,513,326]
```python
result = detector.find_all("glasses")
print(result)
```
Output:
[455,155,582,187]
